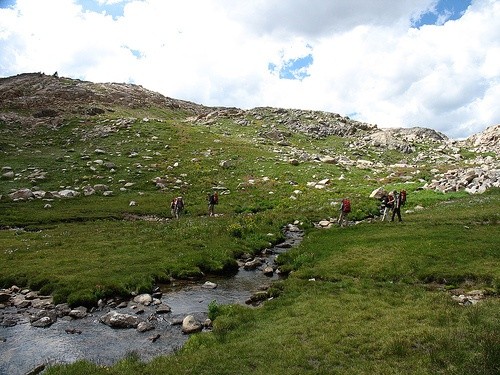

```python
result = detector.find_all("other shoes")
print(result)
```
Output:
[398,220,403,223]
[388,220,393,223]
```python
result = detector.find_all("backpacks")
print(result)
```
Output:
[176,196,184,210]
[388,191,395,209]
[400,189,407,205]
[212,191,218,205]
[342,198,351,213]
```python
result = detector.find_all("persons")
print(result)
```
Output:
[338,198,349,227]
[377,189,402,223]
[170,198,184,220]
[205,192,214,217]
